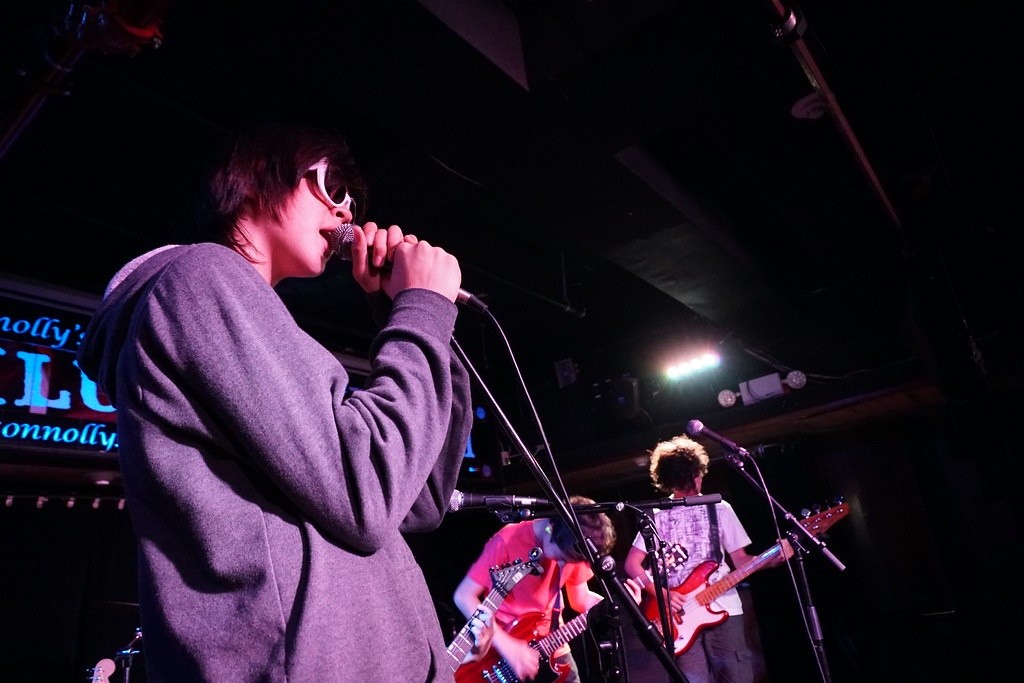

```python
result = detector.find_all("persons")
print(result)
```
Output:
[453,494,617,683]
[623,435,795,682]
[77,117,476,683]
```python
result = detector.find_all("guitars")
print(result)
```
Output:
[455,541,689,683]
[447,545,545,673]
[643,500,849,656]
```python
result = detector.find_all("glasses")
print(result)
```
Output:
[304,164,357,226]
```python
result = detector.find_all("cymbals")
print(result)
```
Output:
[100,600,142,621]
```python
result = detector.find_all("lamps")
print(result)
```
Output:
[717,372,808,408]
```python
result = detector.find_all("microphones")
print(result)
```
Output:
[330,223,488,313]
[686,420,750,457]
[446,489,550,513]
